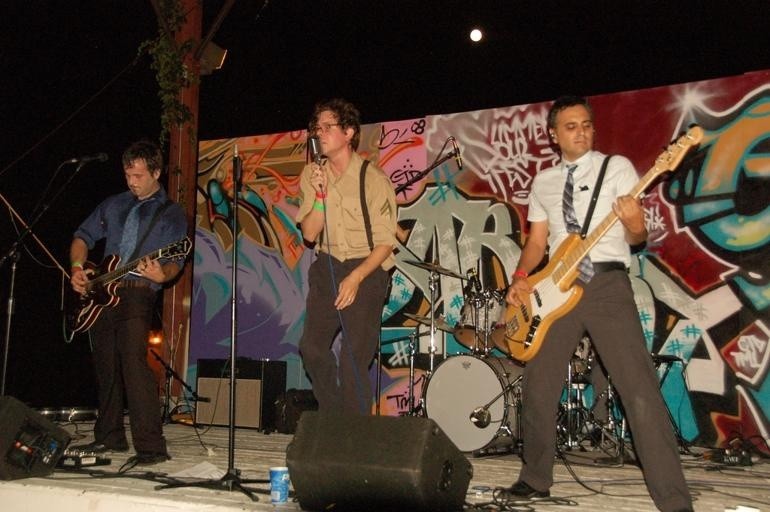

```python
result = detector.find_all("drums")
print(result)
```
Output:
[423,351,523,456]
[454,290,505,353]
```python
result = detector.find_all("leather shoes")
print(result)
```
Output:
[497,482,550,500]
[127,455,166,464]
[71,441,128,452]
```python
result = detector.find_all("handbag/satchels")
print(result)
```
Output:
[273,388,318,434]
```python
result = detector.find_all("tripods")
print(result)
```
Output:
[475,362,700,465]
[154,185,296,502]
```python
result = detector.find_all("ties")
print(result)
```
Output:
[563,166,595,283]
[118,200,145,267]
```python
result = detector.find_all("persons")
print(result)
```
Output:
[495,93,696,507]
[290,100,400,415]
[64,141,182,465]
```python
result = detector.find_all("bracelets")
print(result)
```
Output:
[312,191,327,212]
[511,268,529,285]
[69,258,84,276]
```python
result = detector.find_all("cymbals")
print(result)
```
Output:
[401,258,469,282]
[382,333,435,346]
[403,310,449,331]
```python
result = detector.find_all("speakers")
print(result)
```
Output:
[195,358,287,433]
[0,395,73,481]
[286,412,474,511]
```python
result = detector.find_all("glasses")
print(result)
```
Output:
[309,124,341,135]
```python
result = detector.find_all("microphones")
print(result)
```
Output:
[233,145,242,182]
[309,136,321,165]
[453,140,463,168]
[72,152,109,163]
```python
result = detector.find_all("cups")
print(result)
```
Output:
[270,467,290,503]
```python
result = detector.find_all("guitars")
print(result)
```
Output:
[504,126,702,362]
[62,236,192,342]
